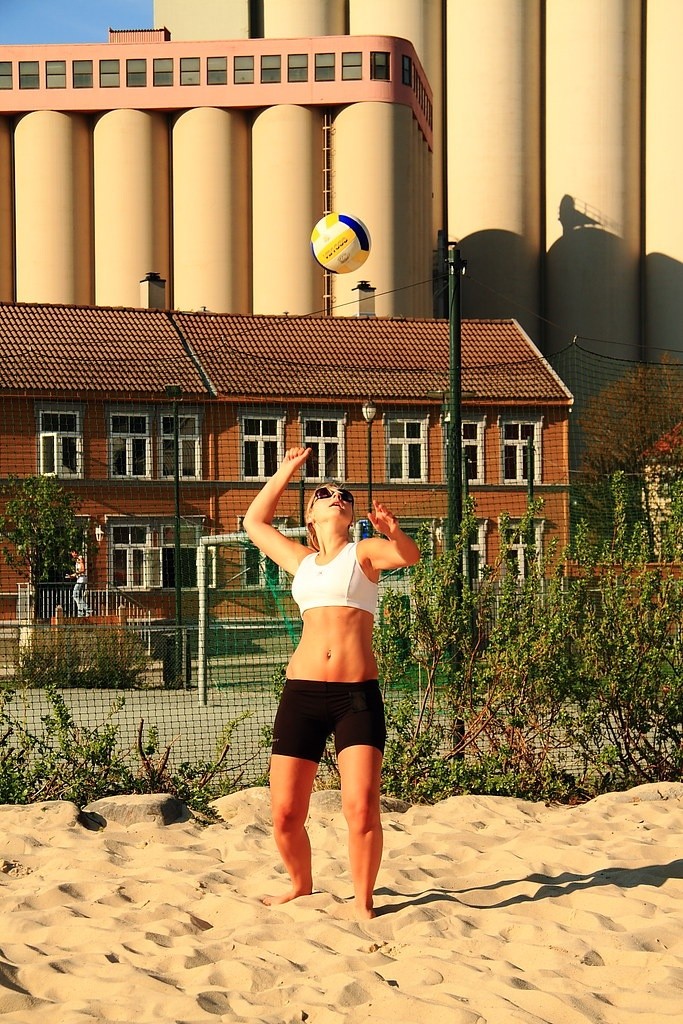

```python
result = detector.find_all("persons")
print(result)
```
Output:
[65,548,94,617]
[242,444,421,922]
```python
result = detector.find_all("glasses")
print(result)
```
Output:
[310,487,354,510]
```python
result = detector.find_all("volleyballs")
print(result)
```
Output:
[310,211,372,273]
